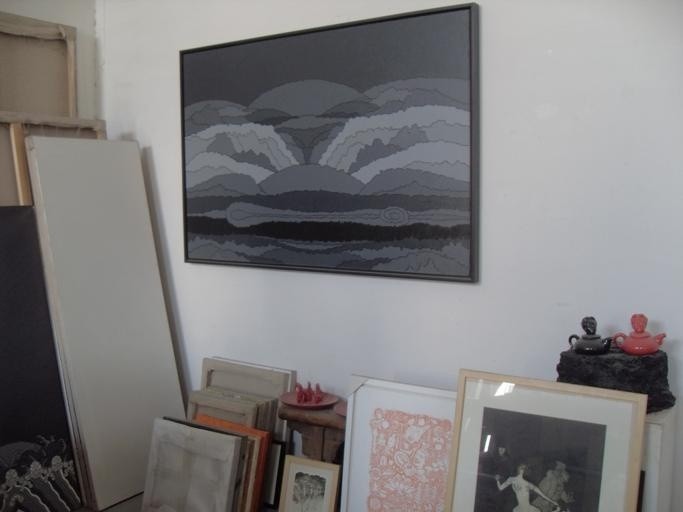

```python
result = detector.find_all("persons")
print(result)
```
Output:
[631,314,648,333]
[581,316,597,334]
[495,460,575,512]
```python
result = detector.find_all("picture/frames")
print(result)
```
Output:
[340,374,459,511]
[142,354,296,511]
[452,369,649,511]
[178,3,482,288]
[279,455,339,511]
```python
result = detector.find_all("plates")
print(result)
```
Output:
[279,389,339,410]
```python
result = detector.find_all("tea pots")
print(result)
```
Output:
[613,312,667,356]
[569,316,612,356]
